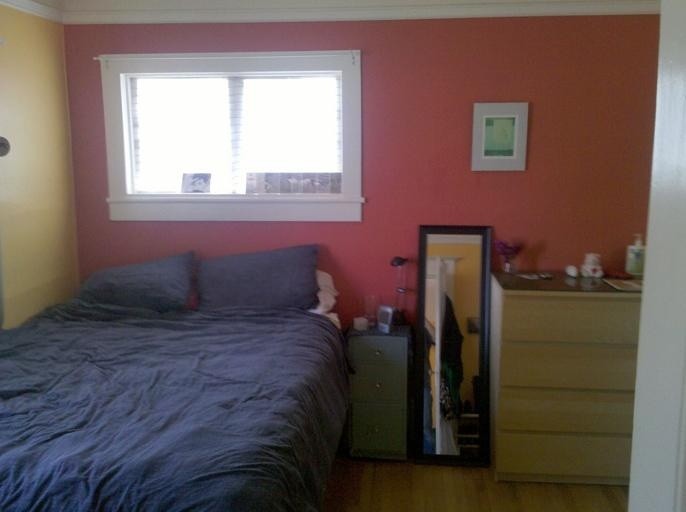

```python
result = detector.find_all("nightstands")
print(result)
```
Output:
[347,324,413,462]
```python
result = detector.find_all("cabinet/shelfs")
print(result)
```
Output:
[489,273,642,488]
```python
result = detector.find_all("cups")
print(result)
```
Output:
[351,293,378,333]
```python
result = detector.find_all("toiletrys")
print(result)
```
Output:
[625,231,646,276]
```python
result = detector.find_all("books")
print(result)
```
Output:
[601,277,643,292]
[243,171,342,194]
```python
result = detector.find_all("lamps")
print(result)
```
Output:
[390,256,409,313]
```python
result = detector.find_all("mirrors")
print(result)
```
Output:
[412,224,493,466]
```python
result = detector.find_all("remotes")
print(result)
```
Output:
[540,271,554,279]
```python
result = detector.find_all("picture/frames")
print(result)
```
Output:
[471,102,530,173]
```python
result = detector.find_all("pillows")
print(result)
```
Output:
[80,243,338,314]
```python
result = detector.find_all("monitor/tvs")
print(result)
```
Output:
[468,318,480,333]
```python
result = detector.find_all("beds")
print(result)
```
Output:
[2,297,341,511]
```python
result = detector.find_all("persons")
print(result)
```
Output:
[182,173,210,192]
[436,290,466,456]
